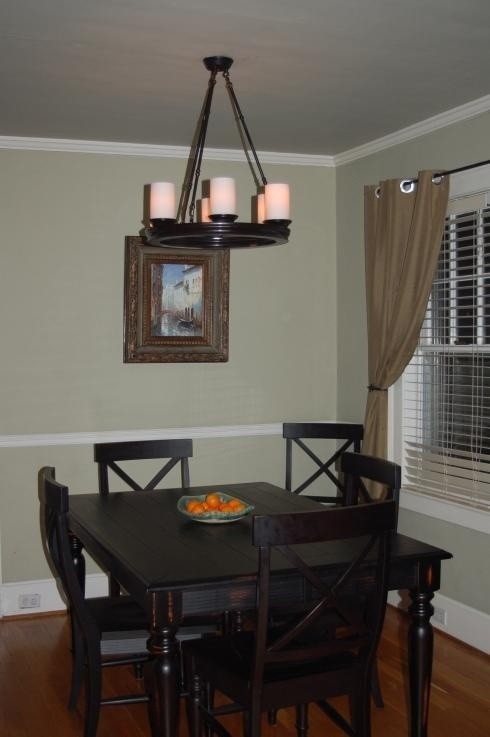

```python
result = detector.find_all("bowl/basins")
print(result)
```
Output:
[176,491,257,524]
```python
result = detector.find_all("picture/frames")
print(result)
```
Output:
[123,236,229,363]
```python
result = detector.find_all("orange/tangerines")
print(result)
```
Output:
[186,494,247,514]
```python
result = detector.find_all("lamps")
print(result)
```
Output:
[145,57,292,251]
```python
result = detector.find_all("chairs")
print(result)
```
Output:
[39,466,210,737]
[267,453,400,724]
[283,423,364,506]
[181,500,397,737]
[94,438,192,678]
